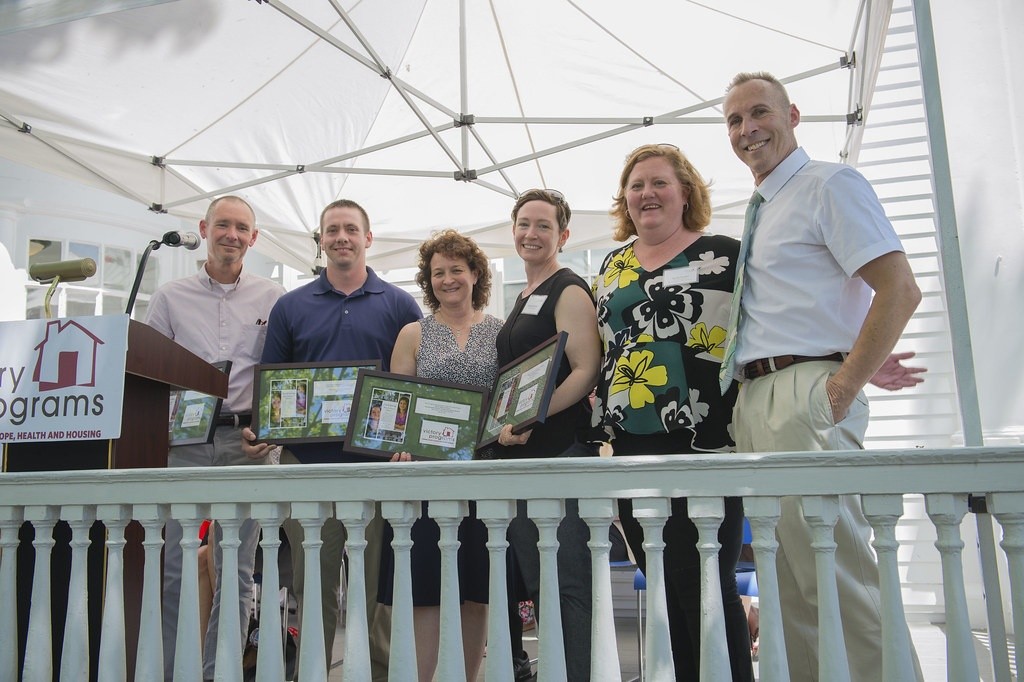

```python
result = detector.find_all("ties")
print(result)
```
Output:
[719,190,762,397]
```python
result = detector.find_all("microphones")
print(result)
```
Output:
[169,232,202,250]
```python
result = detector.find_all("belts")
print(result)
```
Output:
[744,350,850,379]
[215,413,252,426]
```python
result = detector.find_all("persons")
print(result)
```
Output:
[146,73,928,682]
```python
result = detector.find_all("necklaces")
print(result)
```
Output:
[434,306,474,337]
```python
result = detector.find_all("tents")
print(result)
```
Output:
[0,0,897,280]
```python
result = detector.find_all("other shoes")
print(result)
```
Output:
[512,649,531,682]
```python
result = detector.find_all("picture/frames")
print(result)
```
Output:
[343,368,489,460]
[474,330,570,452]
[168,360,234,447]
[249,359,382,447]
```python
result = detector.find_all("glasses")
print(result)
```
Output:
[657,143,680,152]
[518,189,563,201]
